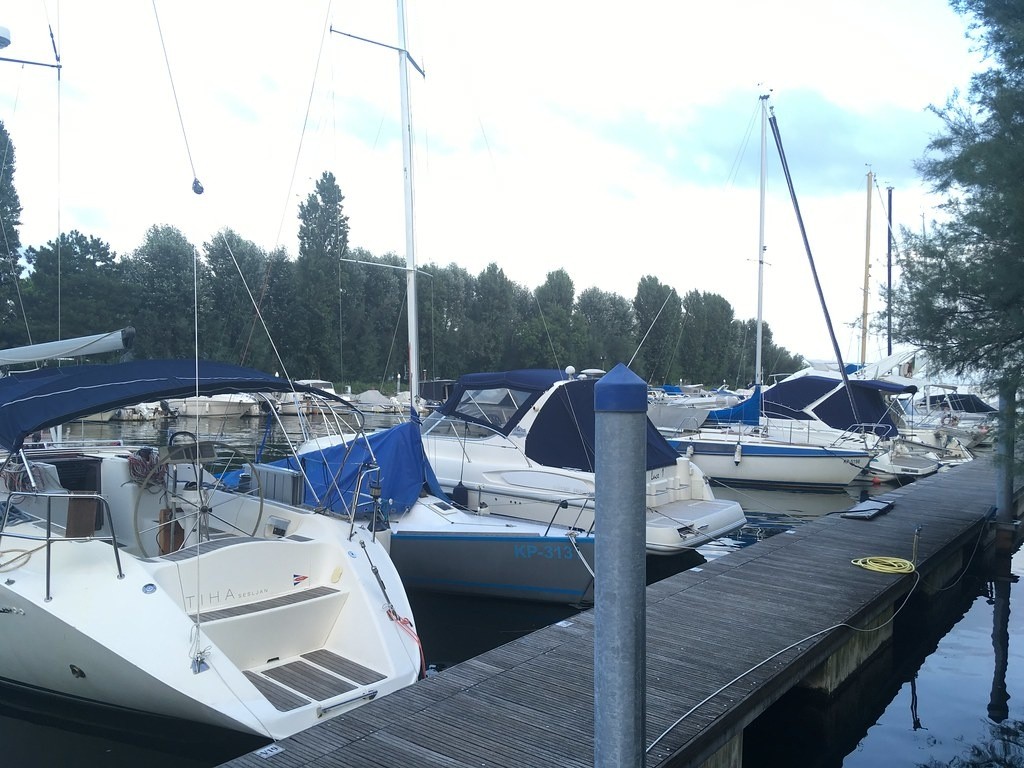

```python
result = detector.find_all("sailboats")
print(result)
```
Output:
[0,0,423,742]
[208,0,749,605]
[644,159,998,475]
[627,89,892,489]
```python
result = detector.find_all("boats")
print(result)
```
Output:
[352,390,404,413]
[280,378,359,415]
[71,401,181,422]
[168,395,255,420]
[390,392,428,413]
[240,392,283,415]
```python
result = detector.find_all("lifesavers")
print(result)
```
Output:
[941,414,959,426]
